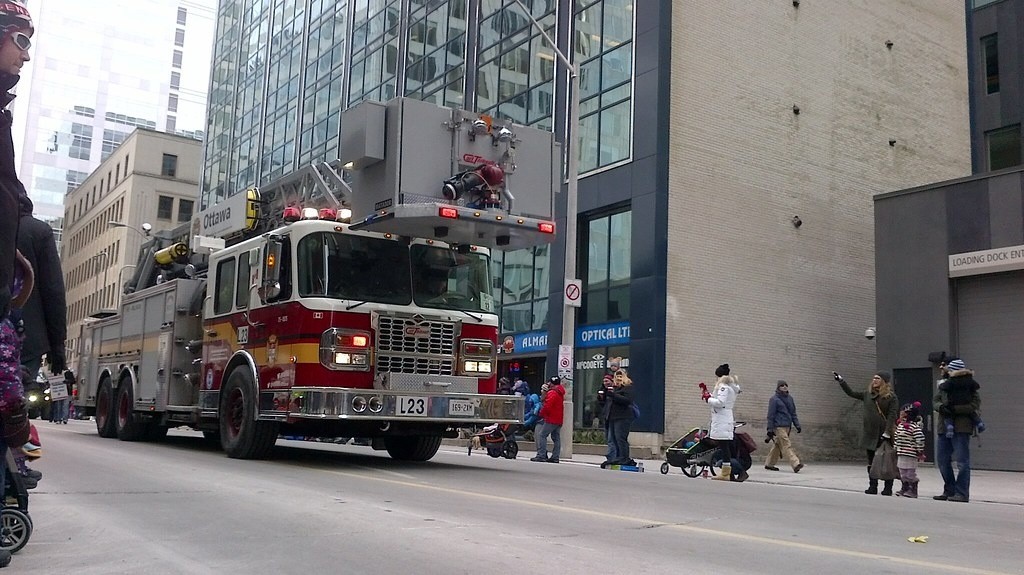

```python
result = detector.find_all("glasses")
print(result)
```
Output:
[780,384,788,387]
[939,364,947,367]
[0,27,31,50]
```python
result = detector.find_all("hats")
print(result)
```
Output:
[876,370,890,383]
[897,402,921,429]
[777,379,788,389]
[943,356,966,372]
[715,364,730,377]
[498,357,620,391]
[0,0,34,39]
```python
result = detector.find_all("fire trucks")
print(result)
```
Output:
[75,96,558,464]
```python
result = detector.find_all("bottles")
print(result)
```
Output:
[639,463,643,473]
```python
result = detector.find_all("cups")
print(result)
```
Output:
[608,387,614,391]
[703,470,708,479]
[598,390,604,400]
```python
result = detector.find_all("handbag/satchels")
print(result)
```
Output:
[631,400,640,419]
[869,442,901,479]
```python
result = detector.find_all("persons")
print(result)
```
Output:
[497,377,531,413]
[432,270,448,291]
[704,363,749,482]
[523,382,551,459]
[0,0,35,568]
[933,357,984,502]
[530,376,565,463]
[598,357,634,461]
[891,402,925,498]
[765,380,803,473]
[833,370,898,496]
[0,198,76,503]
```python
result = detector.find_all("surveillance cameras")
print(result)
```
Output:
[865,330,876,339]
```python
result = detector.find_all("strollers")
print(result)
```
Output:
[0,445,35,554]
[474,422,524,460]
[660,420,747,479]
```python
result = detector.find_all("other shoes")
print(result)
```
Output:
[711,463,804,482]
[865,423,985,503]
[21,476,37,488]
[530,457,547,462]
[24,468,42,481]
[546,457,559,463]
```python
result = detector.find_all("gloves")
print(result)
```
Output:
[699,383,707,392]
[702,394,711,402]
[833,371,843,383]
[45,339,67,376]
[767,424,802,439]
[879,432,891,441]
[917,453,925,462]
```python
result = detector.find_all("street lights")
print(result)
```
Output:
[92,253,108,309]
